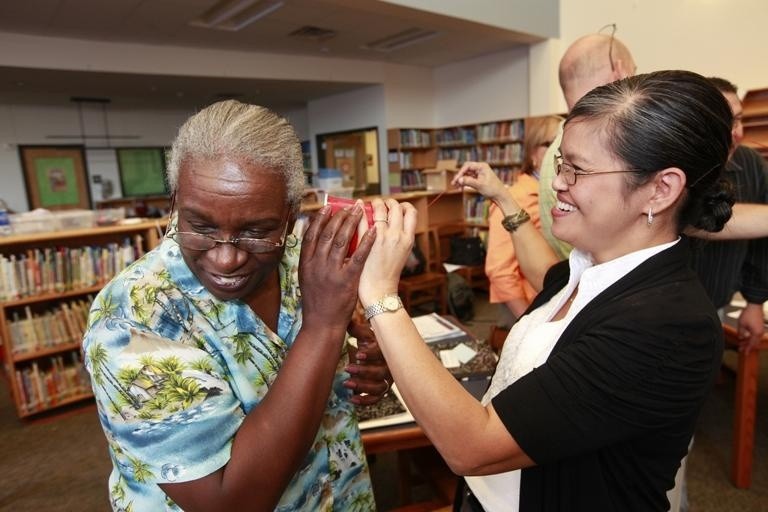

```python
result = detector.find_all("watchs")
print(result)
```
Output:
[364,295,402,321]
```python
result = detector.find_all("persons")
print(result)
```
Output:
[688,77,767,355]
[81,101,376,512]
[483,113,566,318]
[541,34,638,263]
[360,70,733,510]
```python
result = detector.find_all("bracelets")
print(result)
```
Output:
[503,210,528,230]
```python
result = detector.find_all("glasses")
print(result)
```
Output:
[553,152,640,185]
[165,192,293,254]
[599,24,616,72]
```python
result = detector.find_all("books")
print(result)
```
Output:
[0,224,163,410]
[397,120,524,242]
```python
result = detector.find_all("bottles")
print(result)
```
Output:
[0,201,12,237]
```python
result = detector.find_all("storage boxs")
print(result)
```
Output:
[422,158,460,191]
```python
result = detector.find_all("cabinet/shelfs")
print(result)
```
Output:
[0,187,466,427]
[388,118,530,292]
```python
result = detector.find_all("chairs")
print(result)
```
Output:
[397,227,448,319]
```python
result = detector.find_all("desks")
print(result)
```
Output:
[717,298,768,491]
[344,315,500,512]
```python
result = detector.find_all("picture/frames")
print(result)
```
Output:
[116,145,170,197]
[18,144,93,209]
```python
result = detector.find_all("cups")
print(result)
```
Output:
[322,188,391,260]
